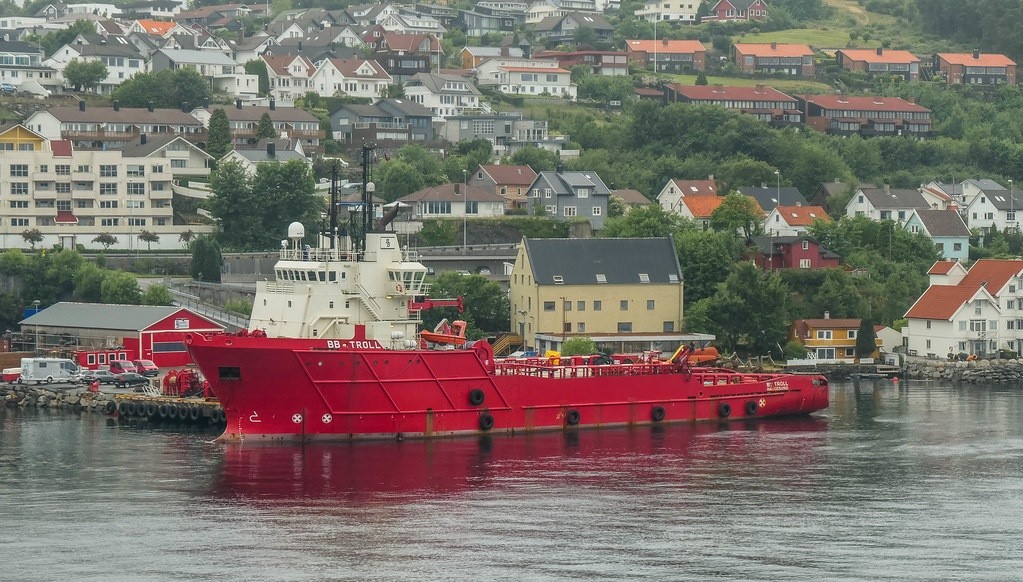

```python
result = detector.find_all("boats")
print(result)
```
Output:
[185,316,830,443]
[420,320,468,345]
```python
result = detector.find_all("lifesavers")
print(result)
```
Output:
[470,390,485,405]
[104,401,116,414]
[719,403,731,417]
[118,402,202,421]
[397,285,403,293]
[567,409,580,424]
[480,414,493,431]
[651,405,666,420]
[211,409,227,423]
[747,400,758,415]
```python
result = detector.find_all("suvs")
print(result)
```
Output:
[113,373,150,388]
[85,370,115,384]
[78,370,89,384]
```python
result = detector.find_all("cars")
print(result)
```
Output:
[0,82,17,93]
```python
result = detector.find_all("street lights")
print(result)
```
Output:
[129,179,133,251]
[33,300,41,353]
[463,169,467,255]
[775,170,781,207]
[889,194,897,261]
[1007,179,1014,233]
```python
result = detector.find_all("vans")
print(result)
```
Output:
[2,367,21,384]
[109,360,138,374]
[134,359,160,377]
[20,357,80,384]
